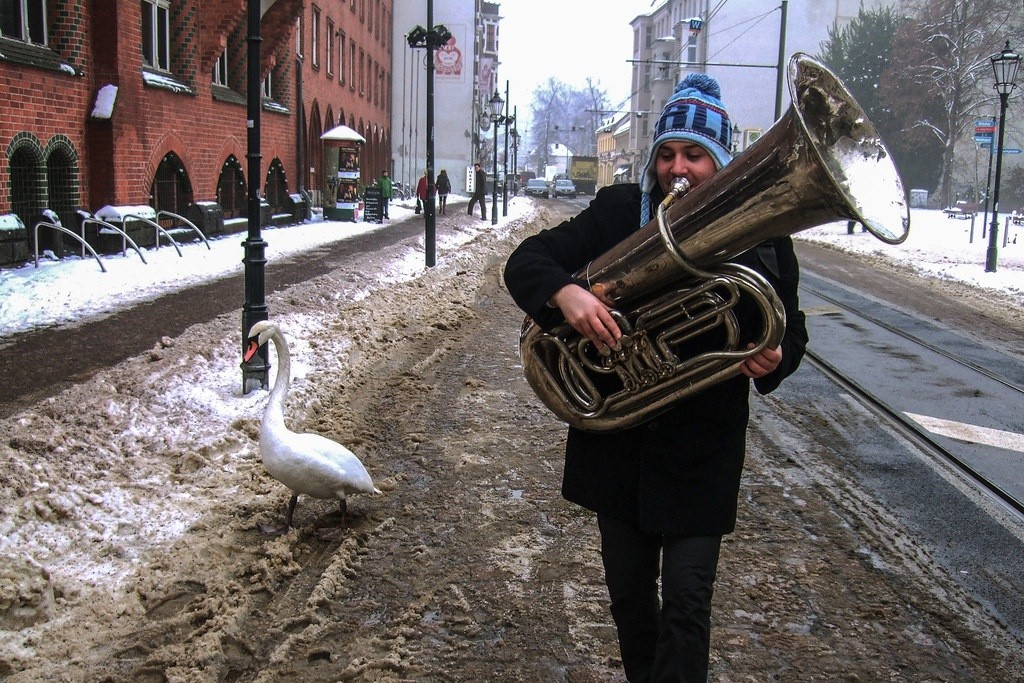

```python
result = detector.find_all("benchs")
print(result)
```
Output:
[945,200,977,219]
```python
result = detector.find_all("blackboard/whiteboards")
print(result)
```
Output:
[363,187,383,224]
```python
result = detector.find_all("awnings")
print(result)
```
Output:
[613,167,628,177]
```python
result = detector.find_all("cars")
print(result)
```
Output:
[522,178,550,199]
[551,172,577,199]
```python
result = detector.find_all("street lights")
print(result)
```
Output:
[488,87,506,225]
[509,104,520,196]
[983,38,1024,272]
[498,80,515,217]
[405,0,452,268]
[731,123,741,153]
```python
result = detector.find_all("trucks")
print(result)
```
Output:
[565,155,599,195]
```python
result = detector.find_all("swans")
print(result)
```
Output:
[242,319,383,541]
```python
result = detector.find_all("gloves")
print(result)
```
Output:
[390,198,392,202]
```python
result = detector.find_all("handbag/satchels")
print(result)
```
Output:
[415,197,422,214]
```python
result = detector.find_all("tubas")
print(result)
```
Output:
[516,52,914,433]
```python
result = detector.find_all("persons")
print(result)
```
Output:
[347,153,358,171]
[416,169,437,218]
[468,163,488,221]
[377,170,393,219]
[435,170,451,215]
[345,184,357,201]
[504,73,809,683]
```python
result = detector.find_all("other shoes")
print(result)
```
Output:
[385,216,389,219]
[439,208,442,213]
[443,213,446,215]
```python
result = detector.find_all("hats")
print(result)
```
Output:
[638,74,733,229]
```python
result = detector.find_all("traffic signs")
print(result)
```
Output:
[975,119,1021,154]
